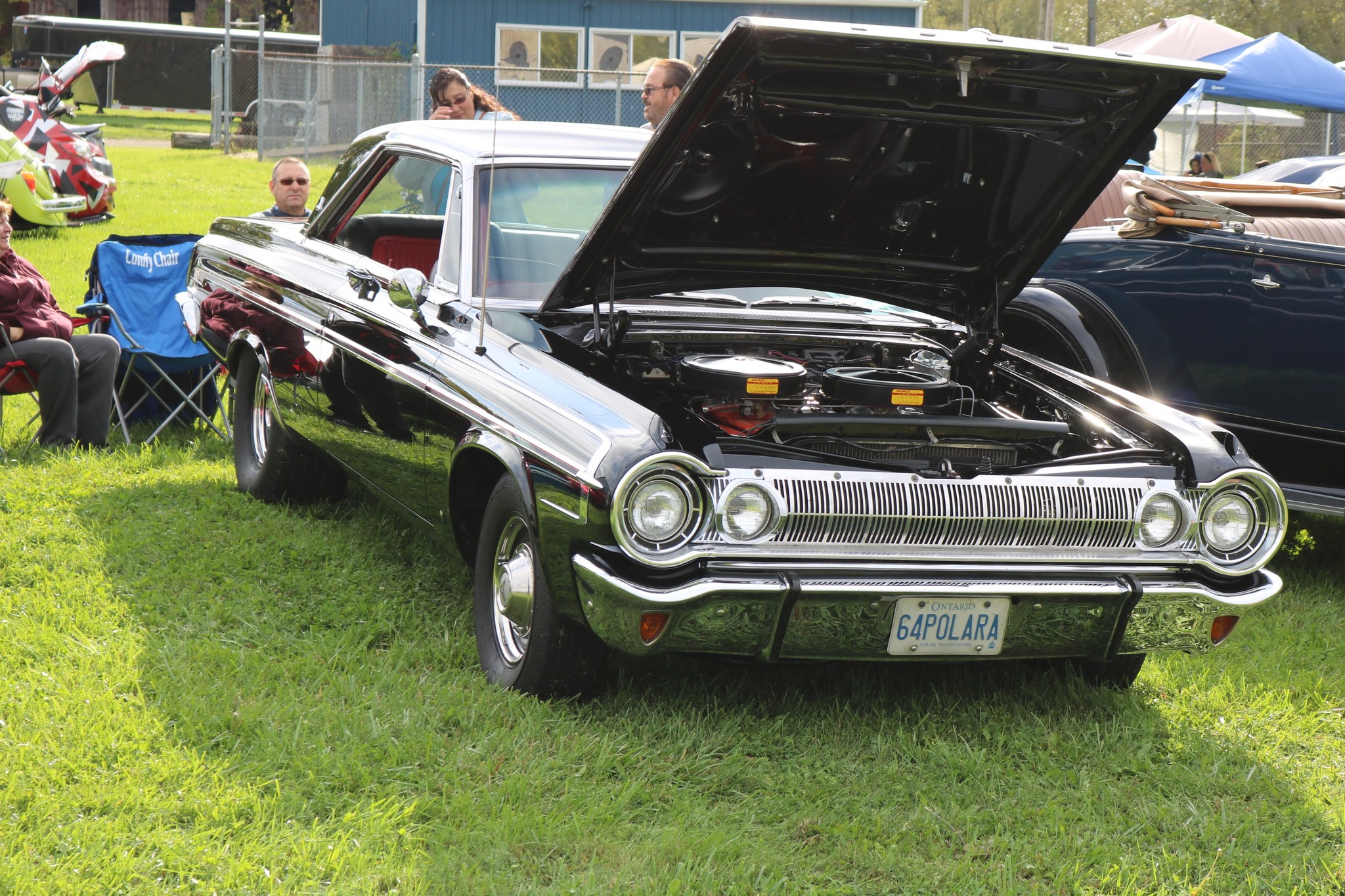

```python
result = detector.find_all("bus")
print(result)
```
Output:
[7,16,321,136]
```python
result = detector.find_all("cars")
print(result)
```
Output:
[1000,155,1345,520]
[176,18,1287,708]
[0,41,125,230]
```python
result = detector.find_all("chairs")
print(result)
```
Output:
[0,233,234,457]
[336,213,506,302]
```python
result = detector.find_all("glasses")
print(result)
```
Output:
[436,86,469,108]
[641,86,673,95]
[275,178,310,185]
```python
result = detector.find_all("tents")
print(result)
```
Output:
[1096,13,1344,177]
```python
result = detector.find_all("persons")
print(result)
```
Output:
[200,278,418,443]
[247,156,314,218]
[637,58,695,133]
[0,200,121,453]
[394,67,541,225]
[1183,152,1224,179]
